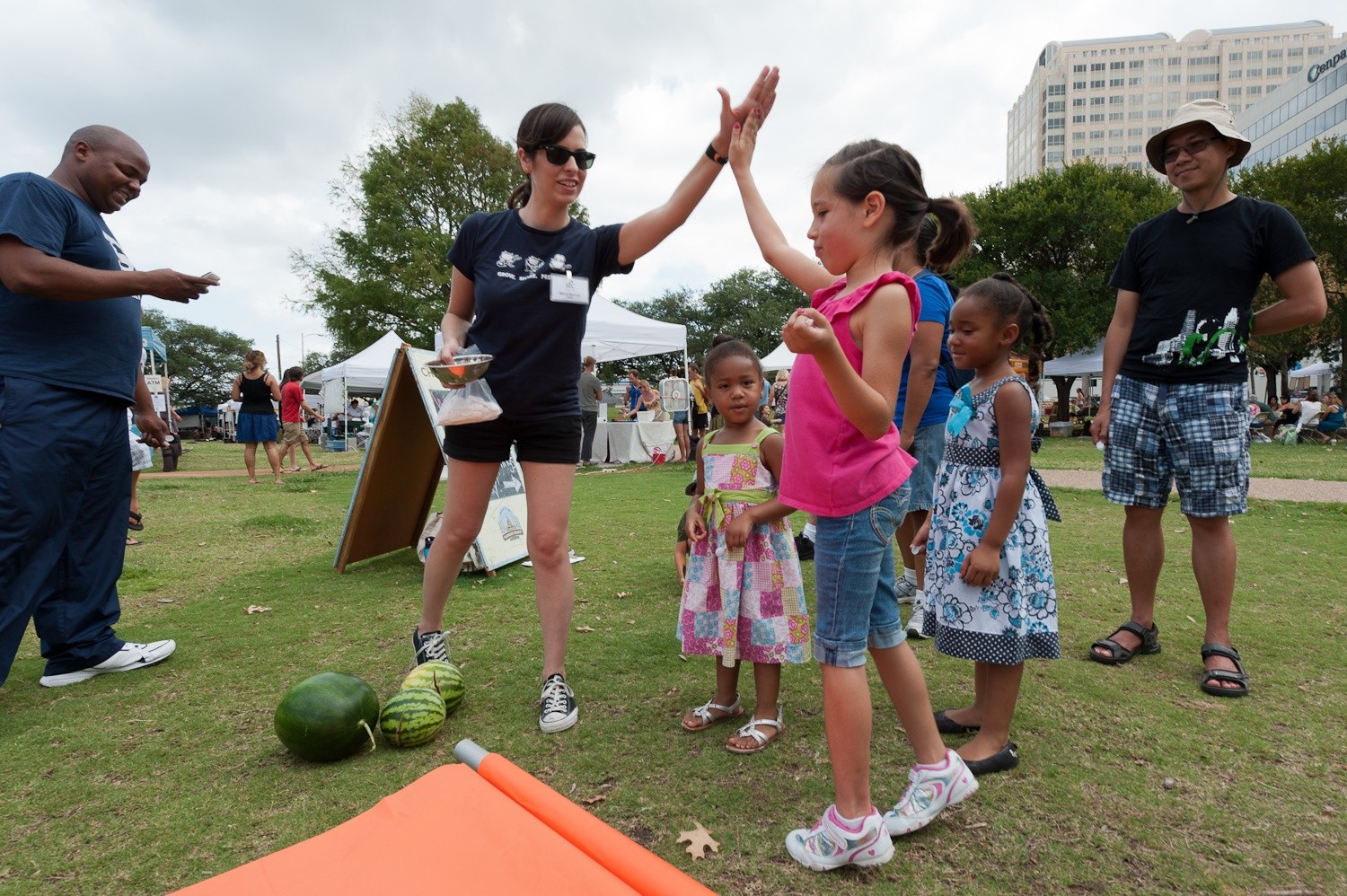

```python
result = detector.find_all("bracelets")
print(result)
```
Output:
[706,143,729,165]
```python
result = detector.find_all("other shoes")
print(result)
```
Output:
[583,461,598,466]
[1321,438,1331,445]
[963,740,1020,776]
[794,532,816,560]
[933,708,978,732]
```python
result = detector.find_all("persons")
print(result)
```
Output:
[0,65,1347,871]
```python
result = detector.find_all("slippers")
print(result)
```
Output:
[311,464,328,472]
[295,467,307,471]
[272,467,292,474]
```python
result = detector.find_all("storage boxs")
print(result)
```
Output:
[1049,422,1073,437]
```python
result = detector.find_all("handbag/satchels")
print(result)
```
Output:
[431,344,505,428]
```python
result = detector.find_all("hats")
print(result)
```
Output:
[1145,98,1251,175]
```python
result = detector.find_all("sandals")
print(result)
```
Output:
[126,538,140,545]
[1089,622,1161,664]
[129,511,144,531]
[681,693,744,731]
[1200,643,1251,696]
[725,706,786,753]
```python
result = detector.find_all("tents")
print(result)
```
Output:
[141,325,171,433]
[1008,351,1042,405]
[760,341,797,372]
[1289,360,1342,396]
[581,290,692,435]
[1040,336,1107,417]
[217,393,323,444]
[174,404,219,433]
[300,330,406,453]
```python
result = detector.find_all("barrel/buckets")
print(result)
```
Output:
[653,452,666,465]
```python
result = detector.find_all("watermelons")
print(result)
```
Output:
[379,688,446,748]
[273,671,380,762]
[401,659,465,716]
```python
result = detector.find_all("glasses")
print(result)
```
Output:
[541,144,597,170]
[639,387,642,389]
[1157,135,1222,164]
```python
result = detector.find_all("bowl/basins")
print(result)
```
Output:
[423,354,494,385]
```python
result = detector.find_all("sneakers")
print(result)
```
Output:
[786,806,894,871]
[905,602,931,640]
[411,626,452,664]
[884,745,980,837]
[39,639,176,687]
[538,673,578,733]
[894,576,916,605]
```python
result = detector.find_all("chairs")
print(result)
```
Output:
[1330,412,1347,441]
[1249,412,1270,443]
[1298,411,1324,445]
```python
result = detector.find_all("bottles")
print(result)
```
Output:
[424,537,434,559]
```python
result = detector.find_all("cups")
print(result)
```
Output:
[1331,439,1337,446]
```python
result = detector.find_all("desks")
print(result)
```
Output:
[590,421,682,464]
